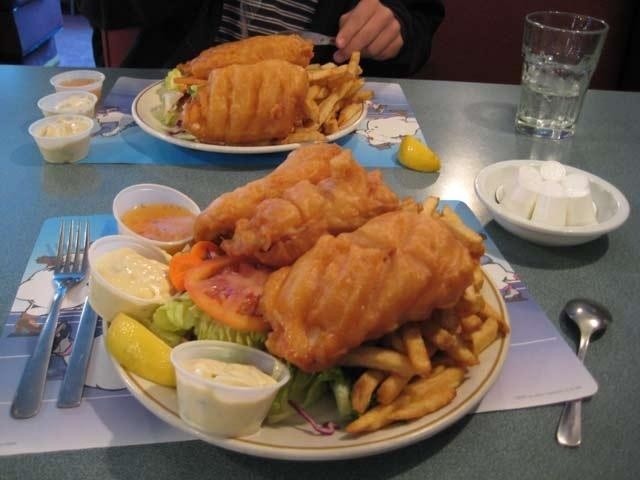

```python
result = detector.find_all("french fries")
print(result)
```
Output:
[281,51,373,144]
[337,195,511,434]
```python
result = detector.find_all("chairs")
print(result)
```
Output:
[100,25,136,71]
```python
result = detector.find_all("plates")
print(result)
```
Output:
[474,158,632,248]
[111,257,511,463]
[131,75,366,157]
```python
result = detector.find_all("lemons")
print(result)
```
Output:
[397,135,441,174]
[107,313,176,390]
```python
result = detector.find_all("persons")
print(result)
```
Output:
[76,0,445,79]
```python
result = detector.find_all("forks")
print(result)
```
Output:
[11,220,87,419]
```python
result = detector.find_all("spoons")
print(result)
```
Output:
[555,297,606,449]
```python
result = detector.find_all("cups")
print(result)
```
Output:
[172,341,290,436]
[28,70,106,165]
[87,179,204,324]
[515,9,608,133]
